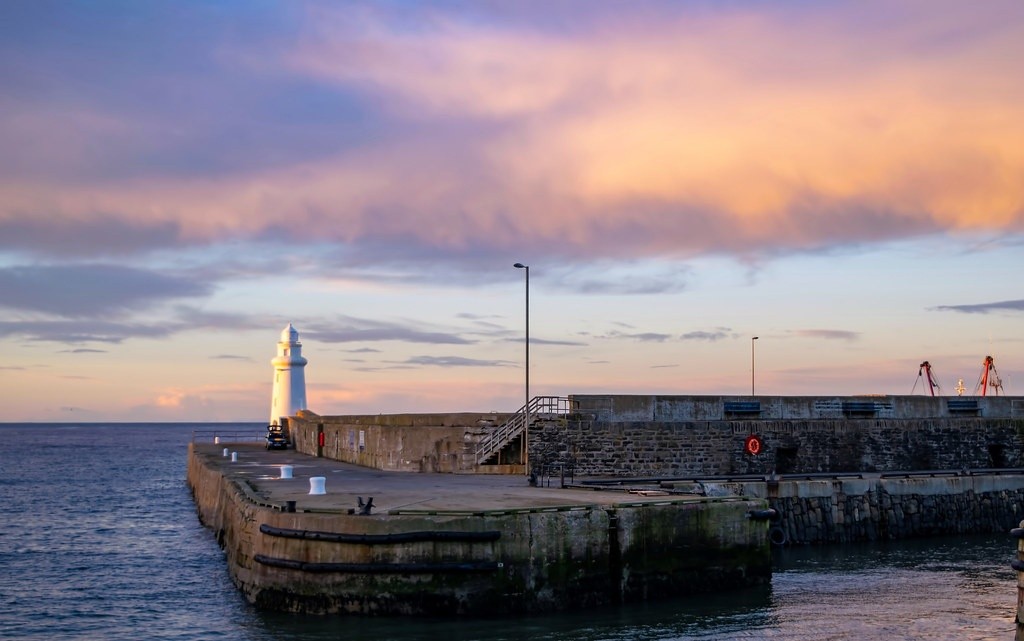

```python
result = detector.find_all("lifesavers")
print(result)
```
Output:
[748,439,759,454]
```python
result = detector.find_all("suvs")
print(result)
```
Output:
[265,425,287,451]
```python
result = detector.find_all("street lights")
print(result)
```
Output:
[751,336,758,396]
[513,262,529,478]
